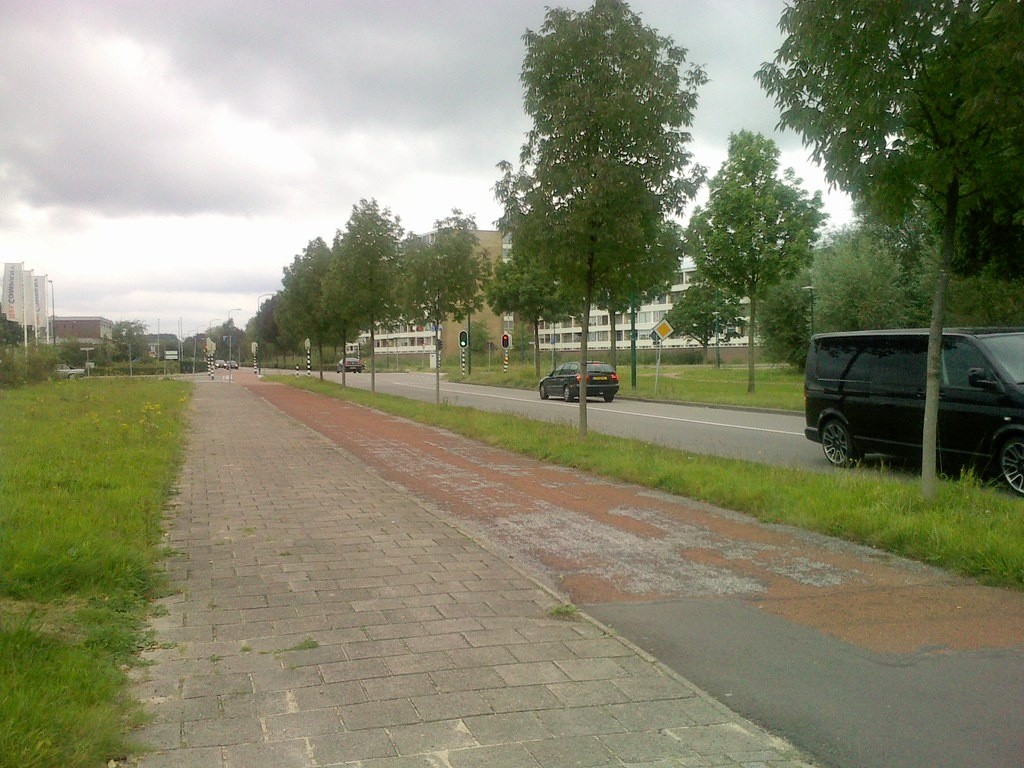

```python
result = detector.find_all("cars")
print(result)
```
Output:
[538,361,620,402]
[215,360,226,369]
[336,357,362,373]
[49,364,85,380]
[224,360,238,370]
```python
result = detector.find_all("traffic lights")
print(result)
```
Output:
[502,334,509,348]
[460,332,466,347]
[255,346,258,352]
[206,338,210,352]
[203,348,207,353]
[439,340,443,350]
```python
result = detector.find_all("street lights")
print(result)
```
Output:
[210,318,221,339]
[47,280,56,346]
[801,282,815,335]
[228,309,242,383]
[156,317,160,362]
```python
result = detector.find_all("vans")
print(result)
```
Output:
[804,328,1023,497]
